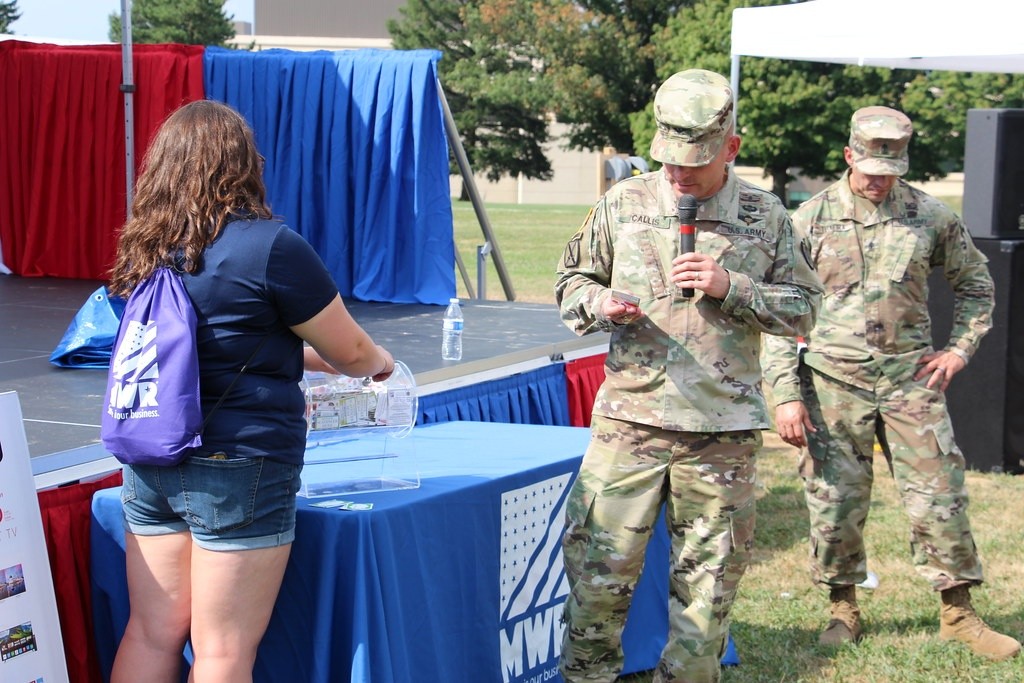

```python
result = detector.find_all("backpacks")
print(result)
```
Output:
[101,213,273,467]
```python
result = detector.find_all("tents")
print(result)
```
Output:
[729,1,1023,136]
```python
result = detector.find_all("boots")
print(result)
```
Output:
[940,588,1021,662]
[820,585,862,649]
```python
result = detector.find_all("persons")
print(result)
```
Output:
[555,69,827,683]
[98,100,396,683]
[758,105,1022,662]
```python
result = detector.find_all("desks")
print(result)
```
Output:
[90,420,740,682]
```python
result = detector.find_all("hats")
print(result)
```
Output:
[650,67,735,167]
[848,105,913,177]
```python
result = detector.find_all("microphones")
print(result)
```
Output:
[678,194,698,297]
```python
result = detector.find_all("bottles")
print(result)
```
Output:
[441,298,464,360]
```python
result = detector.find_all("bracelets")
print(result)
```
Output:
[381,358,387,372]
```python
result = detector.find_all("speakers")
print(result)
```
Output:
[959,107,1024,240]
[926,239,1024,477]
